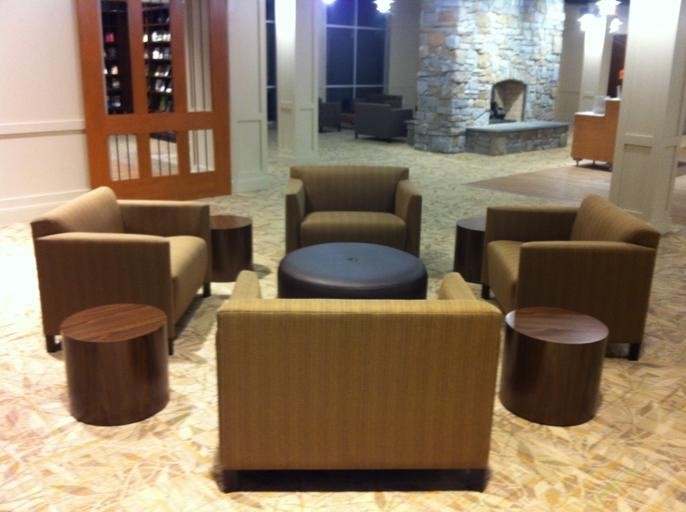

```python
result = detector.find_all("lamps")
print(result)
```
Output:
[373,0,395,14]
[579,13,603,35]
[596,0,622,18]
[609,18,622,33]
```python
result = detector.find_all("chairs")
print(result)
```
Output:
[29,185,211,354]
[571,98,620,168]
[283,163,423,259]
[216,269,503,492]
[318,95,414,142]
[483,194,660,361]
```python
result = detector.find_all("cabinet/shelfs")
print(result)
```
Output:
[77,0,233,198]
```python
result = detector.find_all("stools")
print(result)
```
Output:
[453,216,486,284]
[208,215,253,282]
[503,306,609,428]
[59,303,168,425]
